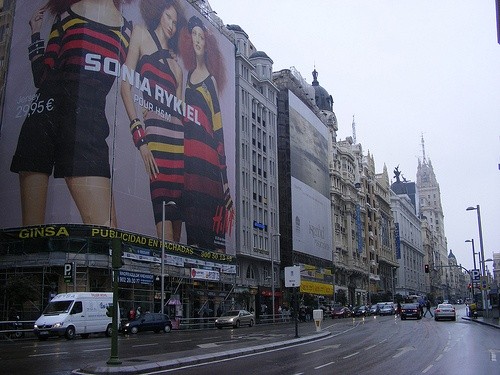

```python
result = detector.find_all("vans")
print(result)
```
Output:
[376,301,394,311]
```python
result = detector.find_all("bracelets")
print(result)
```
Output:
[28,38,44,60]
[130,118,148,150]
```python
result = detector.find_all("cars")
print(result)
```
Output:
[379,304,396,315]
[351,305,369,318]
[330,306,351,319]
[400,304,424,320]
[433,304,456,321]
[394,303,404,314]
[368,305,381,316]
[120,311,173,335]
[214,309,257,330]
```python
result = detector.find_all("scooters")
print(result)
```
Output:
[466,303,478,319]
[0,313,24,339]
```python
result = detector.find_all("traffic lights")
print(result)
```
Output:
[111,237,125,269]
[424,264,429,274]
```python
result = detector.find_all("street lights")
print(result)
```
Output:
[390,266,396,302]
[161,200,177,313]
[270,232,281,323]
[332,249,340,306]
[465,239,477,269]
[465,204,486,276]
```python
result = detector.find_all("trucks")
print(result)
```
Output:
[33,290,114,341]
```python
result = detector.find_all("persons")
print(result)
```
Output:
[192,307,199,323]
[217,307,222,317]
[423,299,433,318]
[397,302,402,314]
[469,302,477,319]
[12,0,136,227]
[281,304,294,322]
[121,1,190,242]
[298,306,313,323]
[177,15,233,252]
[127,308,136,320]
[203,310,214,328]
[136,306,142,319]
